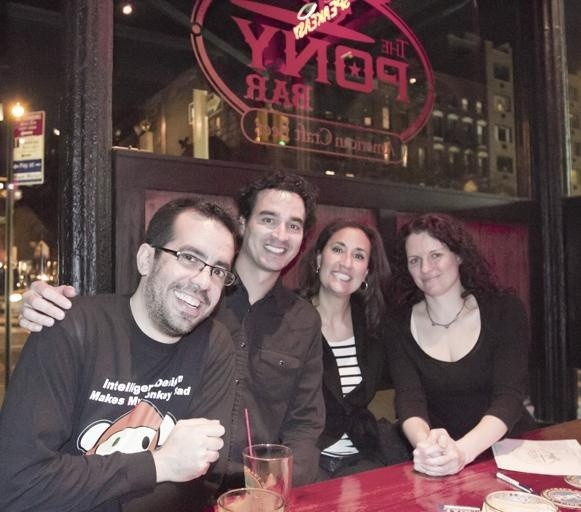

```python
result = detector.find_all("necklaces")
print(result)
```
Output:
[425,297,469,327]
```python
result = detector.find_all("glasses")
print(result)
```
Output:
[151,245,238,289]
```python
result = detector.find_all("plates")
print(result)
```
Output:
[541,475,581,510]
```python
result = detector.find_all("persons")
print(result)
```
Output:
[383,209,542,478]
[293,216,400,486]
[17,161,328,505]
[2,195,246,512]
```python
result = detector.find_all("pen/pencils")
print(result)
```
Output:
[496,472,533,494]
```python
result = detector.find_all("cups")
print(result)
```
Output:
[217,442,294,512]
